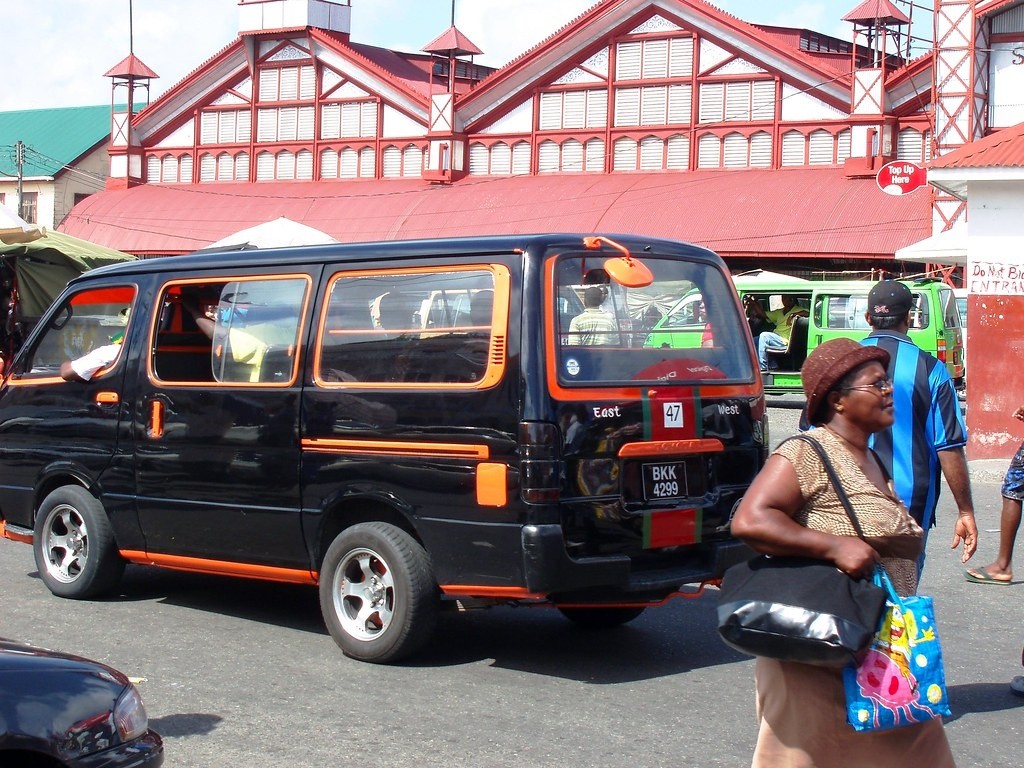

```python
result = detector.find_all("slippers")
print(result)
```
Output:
[964,567,1012,586]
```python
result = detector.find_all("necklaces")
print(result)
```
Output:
[829,427,866,449]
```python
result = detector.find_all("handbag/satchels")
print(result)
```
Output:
[719,553,887,668]
[843,596,955,732]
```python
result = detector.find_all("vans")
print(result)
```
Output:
[413,276,966,393]
[0,238,769,665]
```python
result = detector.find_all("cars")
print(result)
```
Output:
[0,638,165,768]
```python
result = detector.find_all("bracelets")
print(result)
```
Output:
[791,314,795,317]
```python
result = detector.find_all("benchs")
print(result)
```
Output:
[562,344,731,383]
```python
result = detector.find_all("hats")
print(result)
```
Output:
[868,281,919,316]
[802,337,890,425]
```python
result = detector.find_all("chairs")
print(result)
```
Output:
[153,328,216,381]
[766,315,807,371]
[260,344,291,383]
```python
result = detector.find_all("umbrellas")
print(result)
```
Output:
[206,215,342,248]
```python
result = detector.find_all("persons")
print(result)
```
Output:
[730,338,957,768]
[568,287,620,345]
[798,280,978,589]
[699,297,713,346]
[376,294,409,330]
[470,291,494,332]
[963,403,1024,585]
[60,307,133,381]
[180,297,297,382]
[747,294,810,371]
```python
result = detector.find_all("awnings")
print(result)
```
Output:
[56,161,932,262]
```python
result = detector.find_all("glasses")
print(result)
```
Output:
[832,377,893,392]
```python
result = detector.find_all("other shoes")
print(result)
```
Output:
[1010,676,1024,697]
